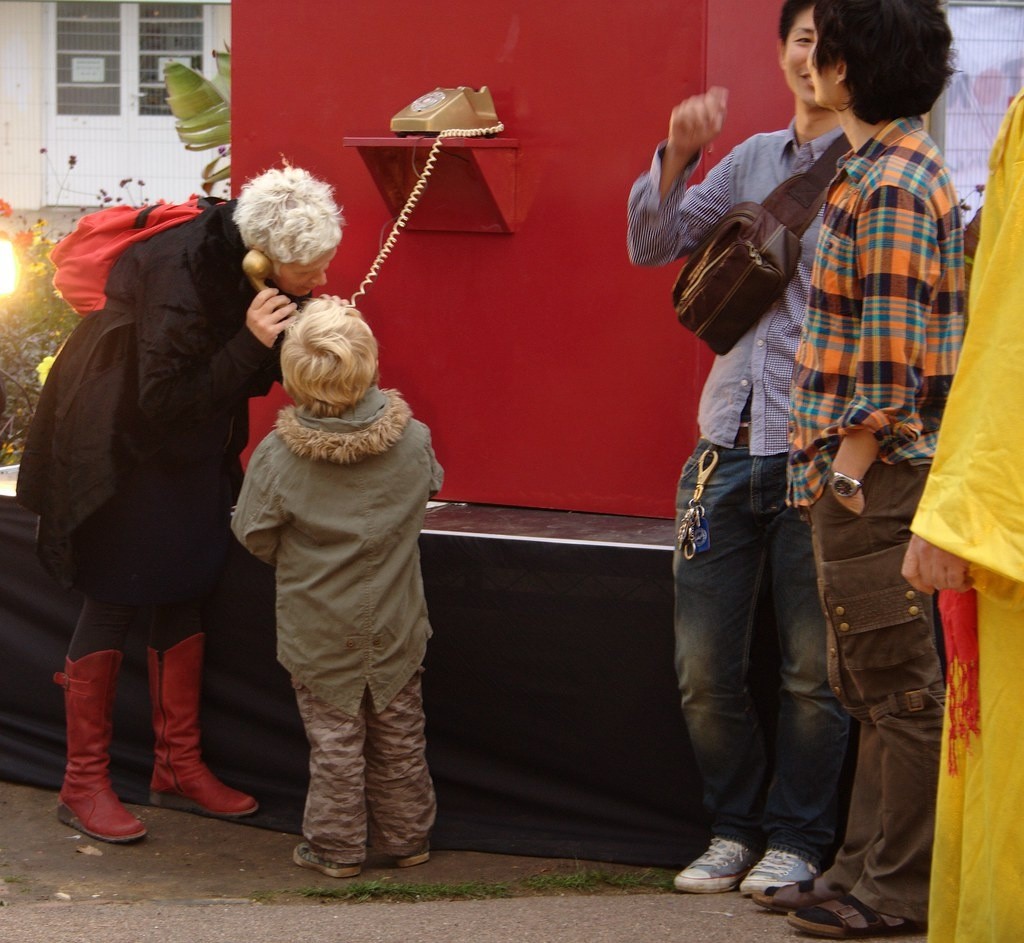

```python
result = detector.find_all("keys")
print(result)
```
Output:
[677,509,692,550]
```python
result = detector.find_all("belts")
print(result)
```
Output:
[733,423,754,448]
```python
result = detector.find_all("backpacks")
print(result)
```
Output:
[50,194,232,317]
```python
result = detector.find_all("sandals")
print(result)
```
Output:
[787,893,928,938]
[752,876,840,913]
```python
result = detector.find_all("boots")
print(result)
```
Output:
[147,633,259,816]
[54,650,147,842]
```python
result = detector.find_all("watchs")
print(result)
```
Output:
[826,470,864,496]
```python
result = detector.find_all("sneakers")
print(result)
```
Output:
[674,836,760,894]
[740,849,820,897]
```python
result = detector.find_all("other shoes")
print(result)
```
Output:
[294,842,361,878]
[394,849,430,866]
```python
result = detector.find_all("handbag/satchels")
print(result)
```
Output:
[672,131,853,356]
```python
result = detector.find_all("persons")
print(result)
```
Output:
[231,298,444,876]
[752,0,966,939]
[17,167,344,842]
[629,0,850,897]
[902,0,1024,943]
